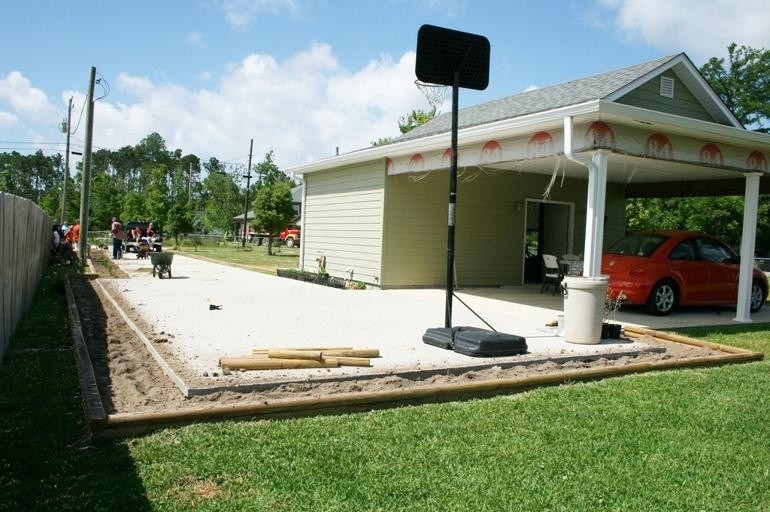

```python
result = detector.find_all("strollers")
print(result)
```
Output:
[134,236,151,259]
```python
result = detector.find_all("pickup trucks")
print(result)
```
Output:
[120,219,164,255]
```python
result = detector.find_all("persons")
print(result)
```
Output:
[111,216,161,259]
[52,219,80,266]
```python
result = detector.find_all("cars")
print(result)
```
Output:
[582,230,767,315]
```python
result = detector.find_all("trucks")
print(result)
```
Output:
[245,223,300,248]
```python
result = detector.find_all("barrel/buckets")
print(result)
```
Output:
[560,277,609,344]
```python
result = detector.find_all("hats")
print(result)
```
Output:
[61,225,69,230]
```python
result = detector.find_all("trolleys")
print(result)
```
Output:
[143,250,176,280]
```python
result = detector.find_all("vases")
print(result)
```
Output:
[610,324,621,340]
[601,324,610,339]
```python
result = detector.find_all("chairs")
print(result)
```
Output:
[540,254,567,296]
[562,254,580,276]
